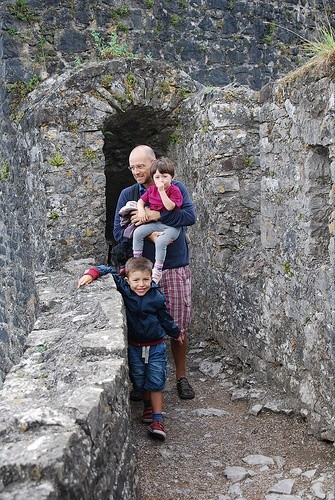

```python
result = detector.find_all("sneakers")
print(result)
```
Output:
[130,388,143,401]
[143,405,153,423]
[177,377,195,399]
[151,269,162,284]
[147,420,166,439]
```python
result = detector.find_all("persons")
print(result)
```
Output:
[131,157,183,284]
[78,257,185,439]
[113,145,197,401]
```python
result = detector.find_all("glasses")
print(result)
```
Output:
[128,161,153,171]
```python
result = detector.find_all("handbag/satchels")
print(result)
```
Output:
[112,238,133,266]
